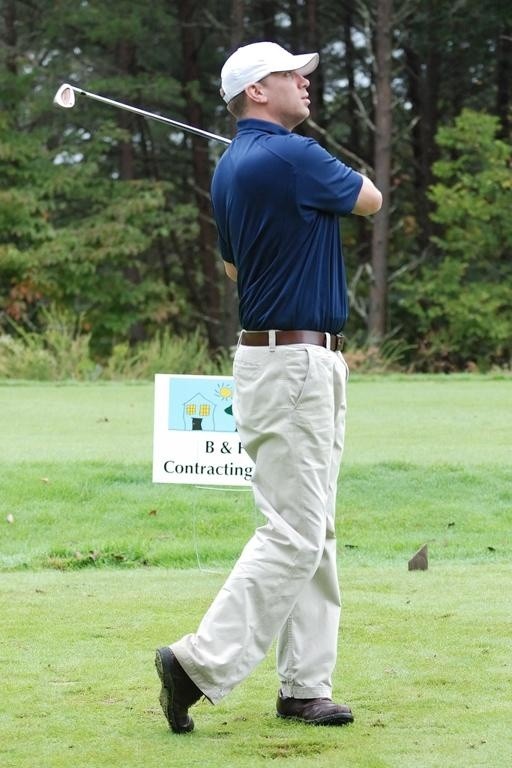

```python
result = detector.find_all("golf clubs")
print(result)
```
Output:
[52,82,230,144]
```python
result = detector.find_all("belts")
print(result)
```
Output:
[240,329,346,352]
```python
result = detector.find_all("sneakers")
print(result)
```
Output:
[274,688,353,724]
[155,646,205,733]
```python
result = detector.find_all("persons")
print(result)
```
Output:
[151,40,384,733]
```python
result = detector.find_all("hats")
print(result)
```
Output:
[220,41,321,102]
